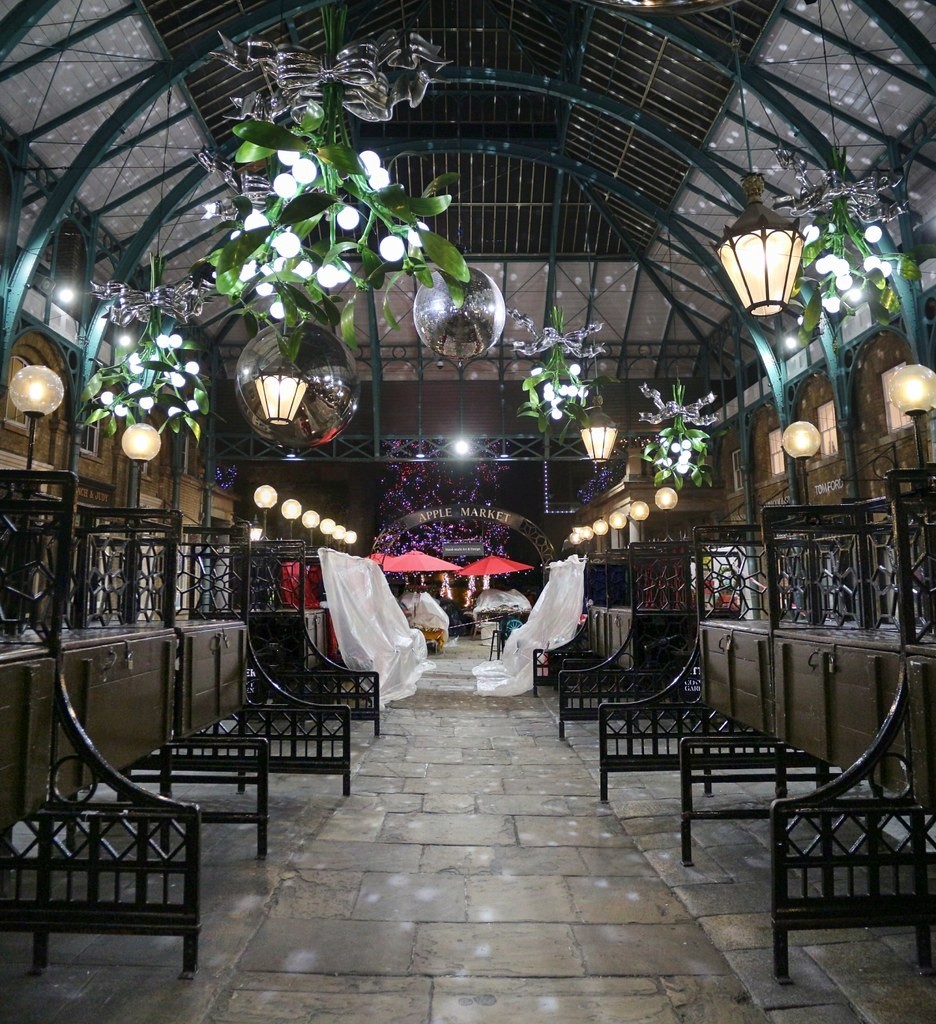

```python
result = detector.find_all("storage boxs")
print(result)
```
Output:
[224,609,325,669]
[773,628,936,796]
[590,605,633,670]
[0,629,178,796]
[0,643,56,832]
[699,620,852,738]
[905,642,936,812]
[66,620,249,740]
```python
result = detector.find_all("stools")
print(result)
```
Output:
[490,630,510,660]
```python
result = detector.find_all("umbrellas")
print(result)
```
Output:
[363,551,393,564]
[382,551,464,591]
[452,554,535,589]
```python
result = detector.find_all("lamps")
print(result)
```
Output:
[320,518,357,554]
[630,501,650,542]
[253,323,311,427]
[10,365,65,498]
[250,514,264,540]
[889,364,936,469]
[782,420,822,505]
[655,487,678,541]
[708,6,810,317]
[609,512,627,549]
[281,499,302,540]
[121,422,162,508]
[302,510,320,546]
[568,518,609,557]
[254,485,278,540]
[580,258,621,465]
